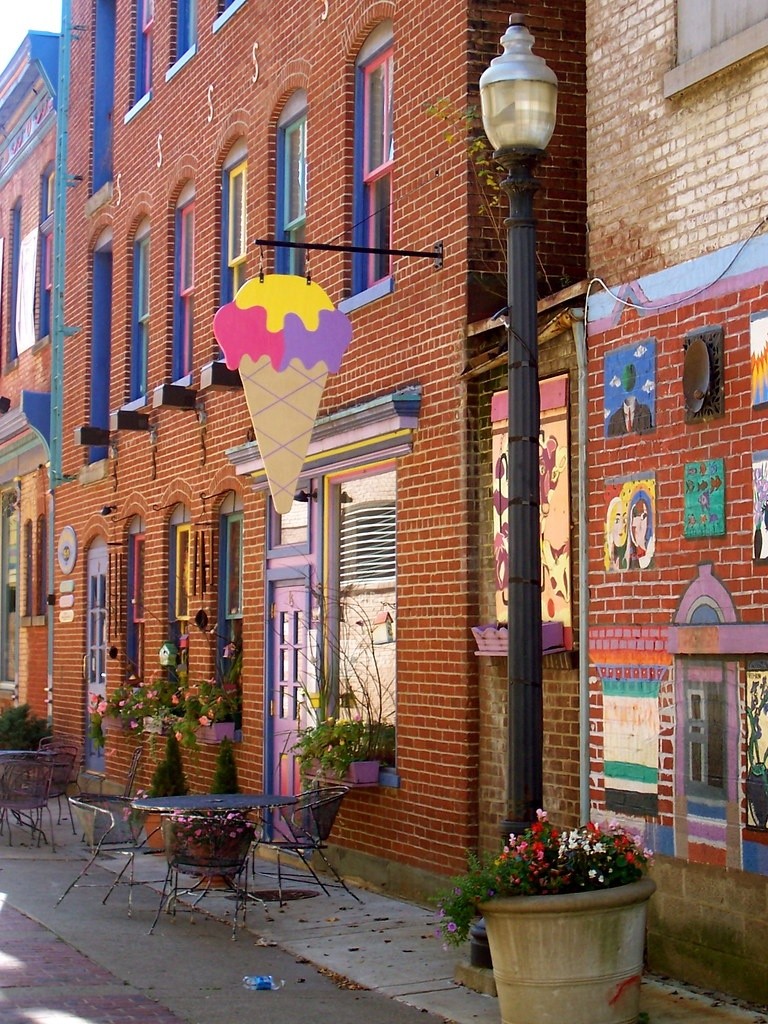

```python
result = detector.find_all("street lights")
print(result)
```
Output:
[469,9,561,968]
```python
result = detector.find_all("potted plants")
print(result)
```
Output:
[196,736,242,888]
[145,733,189,856]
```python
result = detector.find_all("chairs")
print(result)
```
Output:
[148,813,264,942]
[53,794,173,918]
[252,786,365,914]
[0,735,86,847]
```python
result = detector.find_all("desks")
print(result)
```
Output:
[130,794,299,912]
[0,750,58,756]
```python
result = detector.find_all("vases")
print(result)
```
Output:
[180,717,235,745]
[479,879,657,1024]
[472,621,564,652]
[304,757,380,783]
[143,715,175,736]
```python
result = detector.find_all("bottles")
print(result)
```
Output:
[242,976,287,991]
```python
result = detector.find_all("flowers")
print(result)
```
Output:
[88,644,243,778]
[428,809,656,951]
[282,716,395,789]
